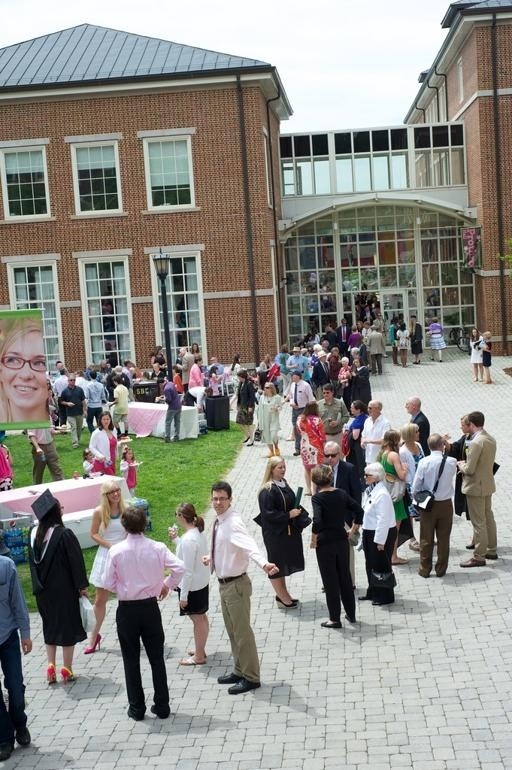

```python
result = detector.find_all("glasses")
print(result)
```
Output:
[323,452,339,458]
[368,407,378,409]
[210,497,230,503]
[323,392,331,394]
[363,472,372,478]
[265,387,271,389]
[106,487,121,496]
[0,355,46,373]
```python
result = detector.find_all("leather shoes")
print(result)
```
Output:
[392,540,497,567]
[48,634,260,720]
[0,745,14,761]
[276,594,383,628]
[243,436,250,443]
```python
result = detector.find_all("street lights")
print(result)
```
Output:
[153,247,170,387]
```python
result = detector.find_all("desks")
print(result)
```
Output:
[0,474,131,521]
[126,401,200,442]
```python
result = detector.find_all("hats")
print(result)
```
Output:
[292,347,302,352]
[31,489,57,521]
[317,350,327,359]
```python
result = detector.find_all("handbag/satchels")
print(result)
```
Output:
[369,569,394,602]
[382,476,405,504]
[412,490,434,512]
[254,428,262,441]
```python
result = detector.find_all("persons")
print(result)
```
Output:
[228,354,260,447]
[0,554,32,762]
[0,324,49,424]
[28,343,223,499]
[0,440,13,491]
[427,318,447,362]
[29,501,90,686]
[200,482,280,695]
[262,296,423,411]
[172,504,210,665]
[258,372,497,628]
[102,506,184,721]
[83,480,130,655]
[469,329,492,384]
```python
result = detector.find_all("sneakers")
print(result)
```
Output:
[293,449,300,455]
[275,448,279,455]
[267,451,275,458]
[16,727,30,747]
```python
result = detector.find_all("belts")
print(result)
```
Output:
[218,572,246,583]
[326,431,342,435]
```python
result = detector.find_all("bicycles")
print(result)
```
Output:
[458,328,471,356]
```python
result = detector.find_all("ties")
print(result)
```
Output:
[295,384,297,403]
[329,469,334,487]
[210,518,218,574]
